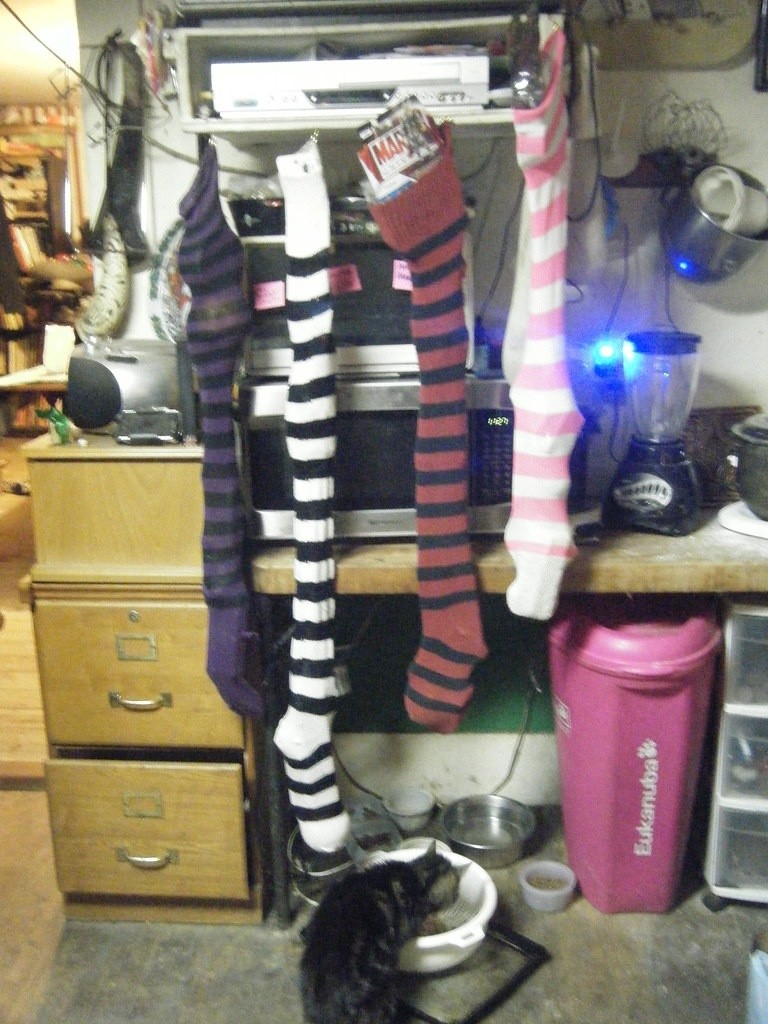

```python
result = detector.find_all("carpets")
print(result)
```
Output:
[35,803,767,1023]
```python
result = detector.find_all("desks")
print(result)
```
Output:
[241,500,767,595]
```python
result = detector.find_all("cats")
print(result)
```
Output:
[301,839,471,1024]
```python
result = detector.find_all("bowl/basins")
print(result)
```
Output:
[382,790,435,832]
[361,849,498,973]
[227,198,283,235]
[440,794,535,867]
[519,861,575,912]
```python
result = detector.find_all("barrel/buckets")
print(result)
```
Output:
[660,163,768,288]
[545,609,720,909]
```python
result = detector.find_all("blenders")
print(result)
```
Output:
[602,332,705,536]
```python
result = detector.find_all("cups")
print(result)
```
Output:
[43,326,75,375]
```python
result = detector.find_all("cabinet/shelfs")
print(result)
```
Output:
[21,435,268,929]
[694,595,768,917]
[0,138,69,438]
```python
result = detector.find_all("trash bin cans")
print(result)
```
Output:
[545,605,722,916]
